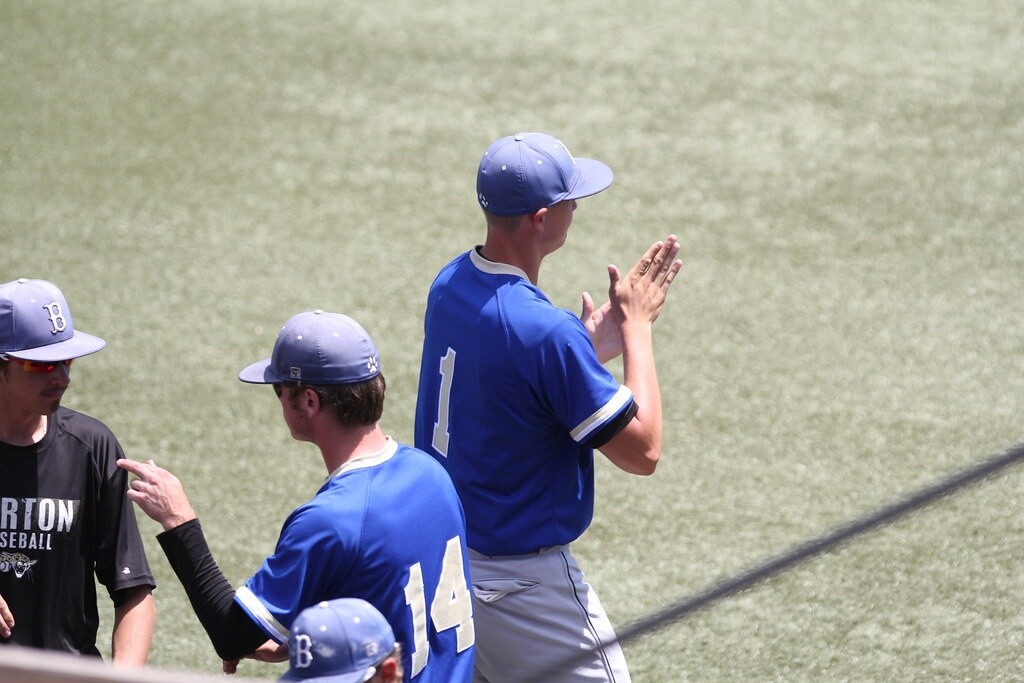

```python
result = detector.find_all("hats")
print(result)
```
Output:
[238,309,380,387]
[277,597,396,682]
[475,131,614,215]
[0,278,107,363]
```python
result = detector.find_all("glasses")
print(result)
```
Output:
[6,355,75,373]
[272,382,329,398]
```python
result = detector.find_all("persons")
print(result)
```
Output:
[0,278,155,669]
[116,308,476,683]
[416,131,683,682]
[272,597,405,683]
